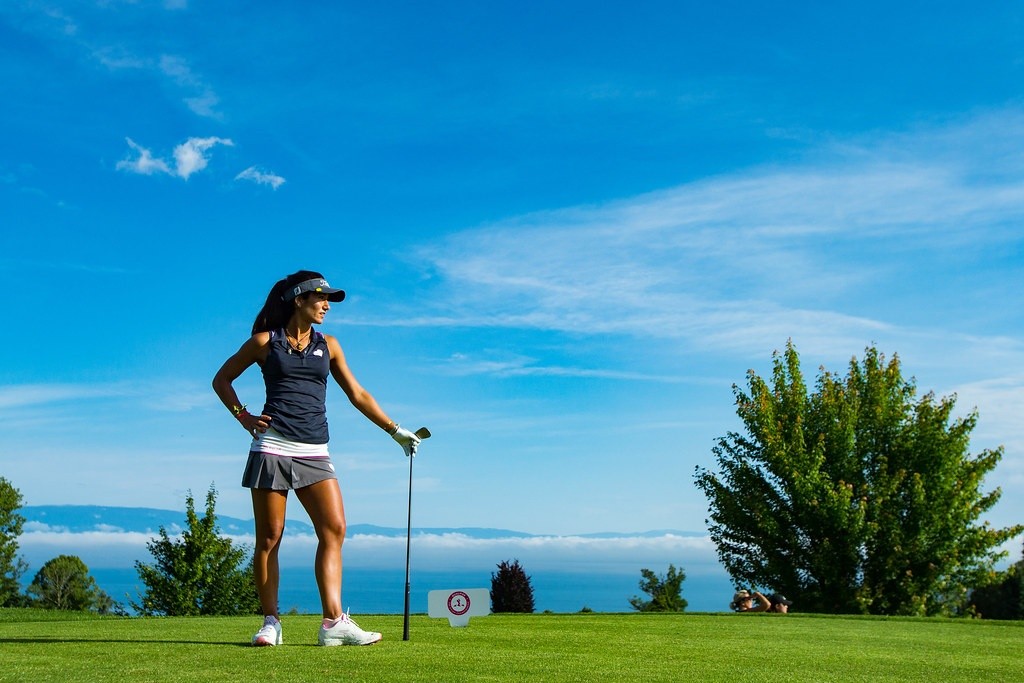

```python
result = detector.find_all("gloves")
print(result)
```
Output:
[389,425,421,457]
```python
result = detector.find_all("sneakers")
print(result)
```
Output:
[251,616,284,646]
[318,607,382,647]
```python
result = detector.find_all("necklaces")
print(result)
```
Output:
[285,327,312,354]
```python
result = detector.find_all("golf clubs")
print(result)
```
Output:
[402,425,433,642]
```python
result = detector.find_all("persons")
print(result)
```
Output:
[730,589,771,612]
[768,594,792,613]
[210,269,422,646]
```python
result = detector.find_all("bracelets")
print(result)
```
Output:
[232,404,251,422]
[389,422,399,435]
[383,420,392,431]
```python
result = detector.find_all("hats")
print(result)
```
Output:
[282,277,345,303]
[769,594,792,604]
